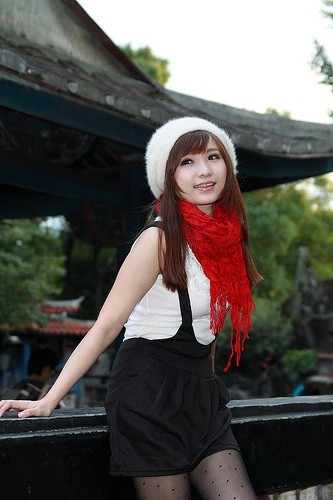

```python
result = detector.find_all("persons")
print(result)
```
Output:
[0,114,259,500]
[14,359,53,401]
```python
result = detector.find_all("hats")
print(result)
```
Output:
[144,117,239,198]
